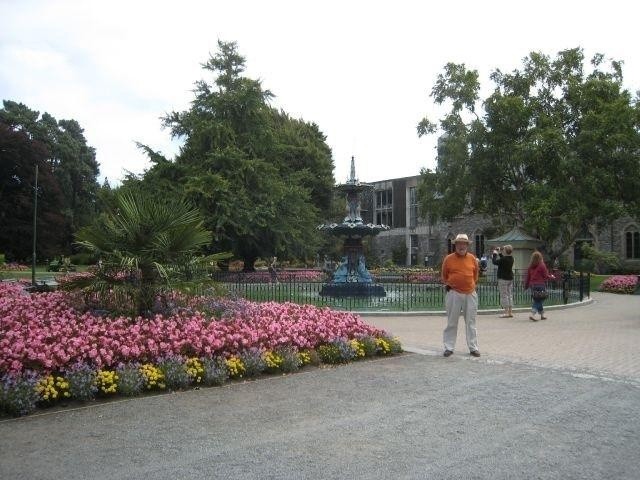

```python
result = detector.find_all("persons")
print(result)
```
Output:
[523,252,558,320]
[441,234,481,356]
[474,252,488,270]
[492,245,514,317]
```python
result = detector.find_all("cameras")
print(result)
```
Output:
[495,248,499,251]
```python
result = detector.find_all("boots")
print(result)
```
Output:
[529,312,548,321]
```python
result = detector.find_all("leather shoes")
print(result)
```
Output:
[471,350,481,357]
[444,350,452,357]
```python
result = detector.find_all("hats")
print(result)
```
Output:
[451,233,475,244]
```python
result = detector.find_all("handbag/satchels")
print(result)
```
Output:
[531,290,551,300]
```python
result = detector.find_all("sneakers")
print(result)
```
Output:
[499,311,515,318]
